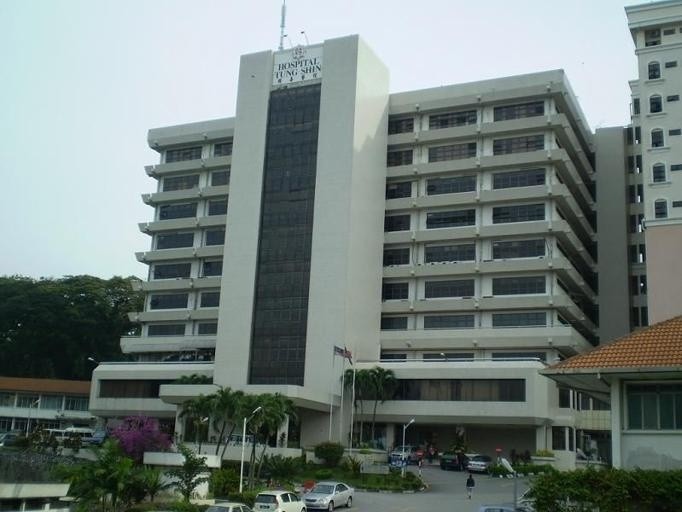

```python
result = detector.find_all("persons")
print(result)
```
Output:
[416,446,423,465]
[466,473,475,500]
[428,443,435,464]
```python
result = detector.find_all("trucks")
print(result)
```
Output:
[0,425,113,448]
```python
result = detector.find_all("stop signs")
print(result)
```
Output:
[495,446,503,453]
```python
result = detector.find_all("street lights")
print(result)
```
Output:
[198,415,211,456]
[401,418,415,478]
[239,406,263,493]
[25,399,43,439]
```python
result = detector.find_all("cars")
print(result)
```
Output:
[479,503,533,512]
[203,480,358,512]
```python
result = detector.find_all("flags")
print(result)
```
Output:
[346,347,354,365]
[335,346,345,356]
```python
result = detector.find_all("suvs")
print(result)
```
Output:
[387,444,494,474]
